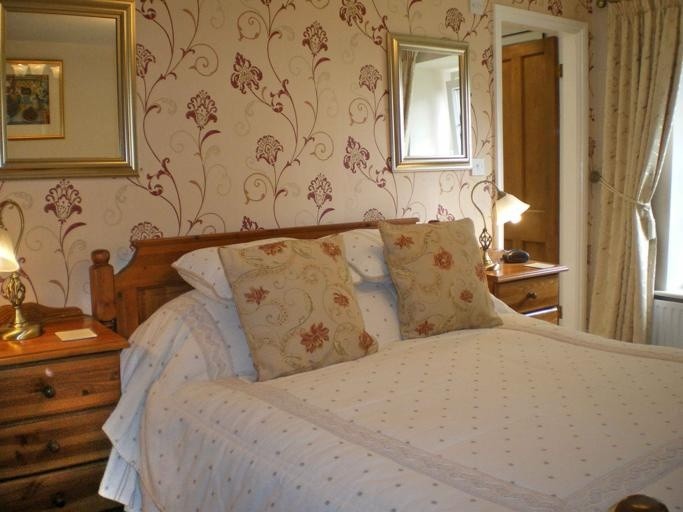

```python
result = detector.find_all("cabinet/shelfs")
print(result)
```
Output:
[6,59,65,139]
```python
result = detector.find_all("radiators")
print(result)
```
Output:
[651,300,683,349]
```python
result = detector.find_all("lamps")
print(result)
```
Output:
[471,180,531,271]
[0,228,42,340]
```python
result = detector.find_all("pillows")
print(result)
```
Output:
[218,228,378,382]
[171,237,362,306]
[320,228,391,283]
[127,282,398,387]
[378,217,504,341]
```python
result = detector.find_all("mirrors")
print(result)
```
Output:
[0,0,138,180]
[386,32,473,173]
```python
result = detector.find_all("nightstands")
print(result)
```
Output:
[483,251,569,326]
[1,303,131,512]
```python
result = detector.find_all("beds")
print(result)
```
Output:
[90,219,683,512]
